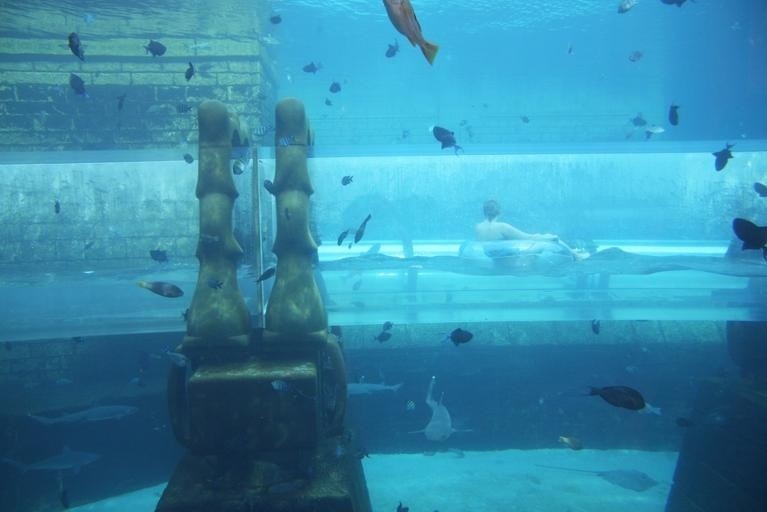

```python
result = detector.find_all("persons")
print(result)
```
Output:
[475,201,558,262]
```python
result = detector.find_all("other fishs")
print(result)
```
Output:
[136,1,501,367]
